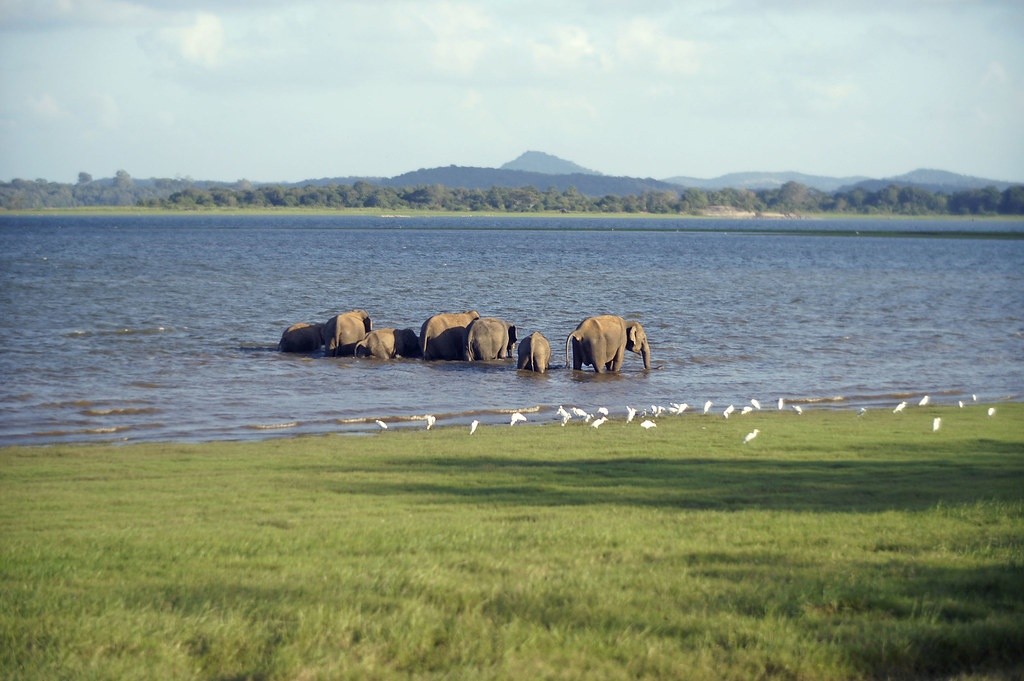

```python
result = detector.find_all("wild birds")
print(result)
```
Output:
[892,401,907,414]
[374,419,388,431]
[857,405,868,417]
[919,396,930,407]
[555,397,804,430]
[427,415,435,429]
[510,412,527,426]
[988,408,995,416]
[959,400,963,408]
[744,428,760,444]
[973,394,976,402]
[470,420,479,435]
[934,417,941,431]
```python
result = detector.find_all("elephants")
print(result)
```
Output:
[417,311,480,362]
[518,331,551,373]
[462,316,518,362]
[567,315,665,375]
[278,321,326,351]
[325,309,373,357]
[352,328,420,362]
